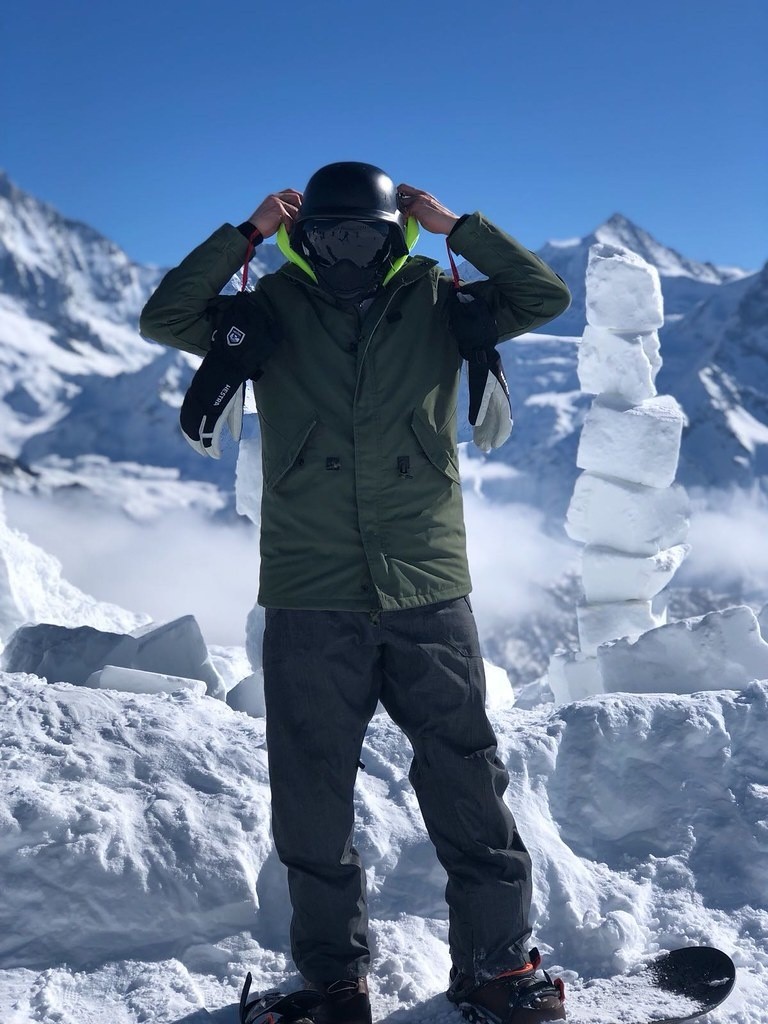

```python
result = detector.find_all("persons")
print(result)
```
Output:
[137,159,574,1024]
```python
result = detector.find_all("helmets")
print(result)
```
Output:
[294,162,409,294]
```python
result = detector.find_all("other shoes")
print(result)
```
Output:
[446,961,567,1024]
[309,973,372,1024]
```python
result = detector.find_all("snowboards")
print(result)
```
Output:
[562,946,736,1024]
